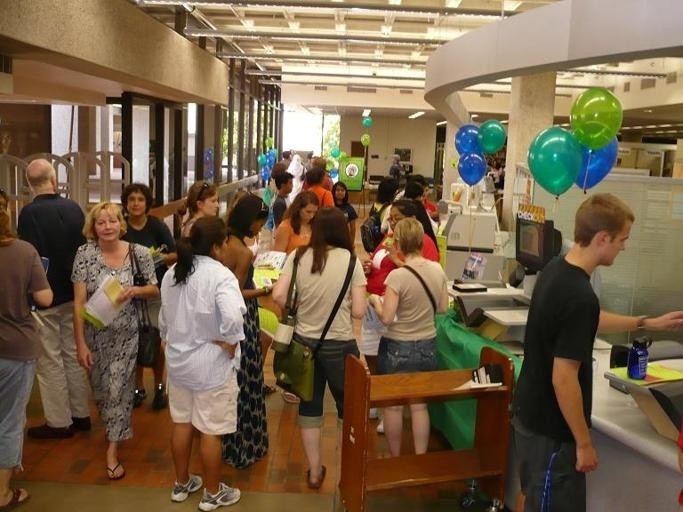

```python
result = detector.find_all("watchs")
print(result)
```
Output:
[637,315,649,331]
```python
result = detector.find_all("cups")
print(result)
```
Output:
[480,193,495,211]
[524,275,538,298]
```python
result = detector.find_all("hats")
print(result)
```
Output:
[391,155,400,160]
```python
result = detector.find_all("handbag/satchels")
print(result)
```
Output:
[128,241,161,367]
[273,250,357,402]
[301,167,305,181]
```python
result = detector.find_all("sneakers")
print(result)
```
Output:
[375,420,384,434]
[370,409,378,419]
[198,483,242,510]
[133,385,147,408]
[152,385,166,412]
[170,475,202,503]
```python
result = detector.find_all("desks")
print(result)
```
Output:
[489,336,683,512]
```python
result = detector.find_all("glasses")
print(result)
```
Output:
[387,217,400,224]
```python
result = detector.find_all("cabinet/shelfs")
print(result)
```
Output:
[339,345,514,512]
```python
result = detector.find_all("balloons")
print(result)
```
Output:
[574,135,619,191]
[360,133,371,147]
[265,137,273,147]
[477,120,506,155]
[261,168,270,180]
[326,160,334,172]
[330,167,338,178]
[360,116,374,128]
[331,147,340,158]
[569,86,623,152]
[257,154,266,165]
[457,151,487,187]
[267,156,275,168]
[454,124,481,156]
[527,126,582,197]
[269,148,276,157]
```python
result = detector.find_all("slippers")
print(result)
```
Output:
[0,487,31,512]
[107,463,126,481]
[281,392,300,403]
[307,466,326,488]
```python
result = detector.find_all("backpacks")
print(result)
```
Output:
[360,202,391,252]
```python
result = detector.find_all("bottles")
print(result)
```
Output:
[627,335,653,379]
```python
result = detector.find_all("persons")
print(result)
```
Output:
[262,163,288,208]
[272,191,320,405]
[422,183,439,222]
[16,158,91,439]
[511,192,683,512]
[393,174,426,202]
[286,154,307,208]
[221,192,273,470]
[70,201,160,481]
[271,206,369,491]
[271,172,294,229]
[389,153,402,187]
[305,167,335,209]
[301,157,334,191]
[120,183,178,410]
[0,190,53,512]
[360,199,441,434]
[178,179,220,238]
[158,215,248,512]
[380,181,439,236]
[369,176,400,252]
[280,151,291,169]
[332,180,358,250]
[369,217,450,456]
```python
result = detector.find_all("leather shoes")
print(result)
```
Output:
[72,416,91,432]
[27,423,74,441]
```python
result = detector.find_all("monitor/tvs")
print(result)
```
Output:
[514,213,562,271]
